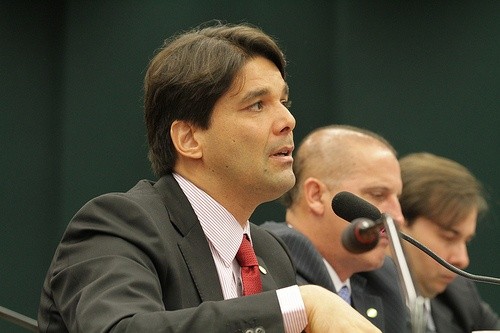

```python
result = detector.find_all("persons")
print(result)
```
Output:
[37,25,382,333]
[358,149,500,333]
[258,124,405,333]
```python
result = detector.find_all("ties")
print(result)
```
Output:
[339,287,351,303]
[236,235,260,296]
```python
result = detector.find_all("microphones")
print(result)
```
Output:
[340,213,434,332]
[332,191,500,286]
